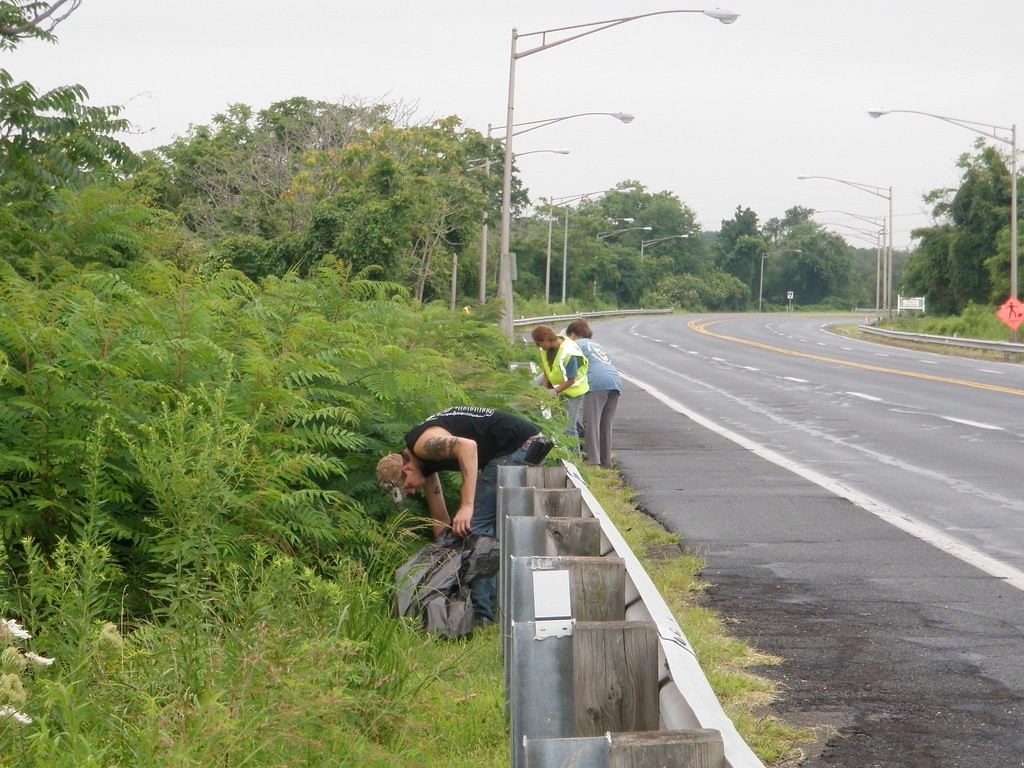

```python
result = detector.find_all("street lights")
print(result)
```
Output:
[479,112,634,307]
[867,109,1019,347]
[759,250,802,312]
[562,187,637,305]
[498,7,742,346]
[449,148,571,312]
[796,175,893,322]
[593,226,654,300]
[815,209,888,322]
[641,233,689,265]
[545,189,631,304]
[815,222,880,313]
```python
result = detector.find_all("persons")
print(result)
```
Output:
[529,318,622,472]
[374,407,552,621]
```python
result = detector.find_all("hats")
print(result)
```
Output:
[377,454,406,504]
